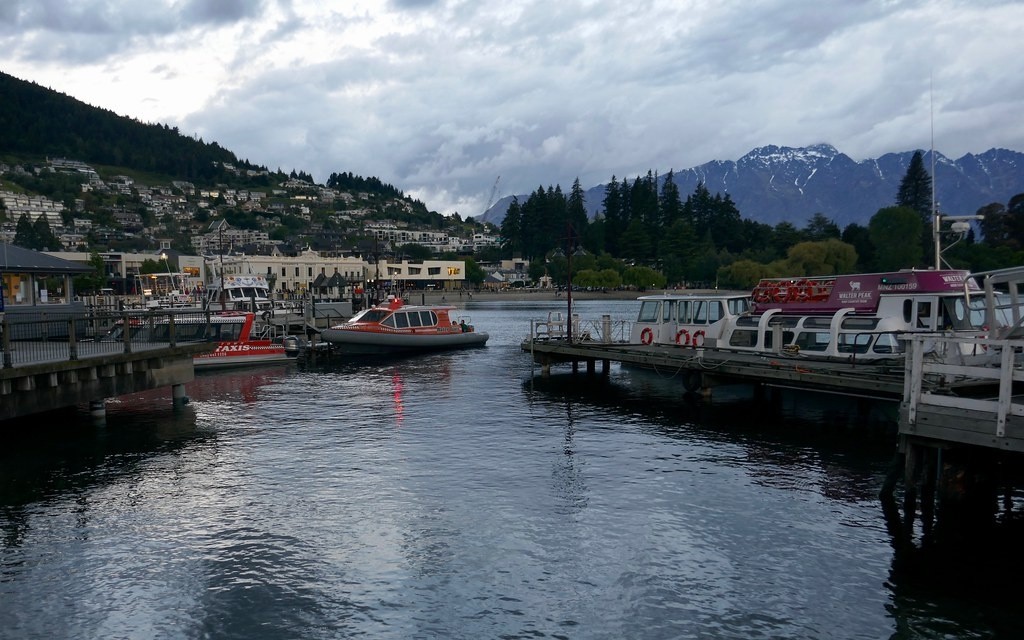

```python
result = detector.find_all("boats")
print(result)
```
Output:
[196,275,306,336]
[321,294,490,350]
[701,267,1024,367]
[94,291,257,344]
[613,293,754,373]
[192,335,300,368]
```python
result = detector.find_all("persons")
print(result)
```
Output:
[276,288,308,300]
[185,287,207,301]
[459,320,467,333]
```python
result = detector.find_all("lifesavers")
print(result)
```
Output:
[676,328,690,345]
[751,279,836,303]
[641,327,653,344]
[692,329,705,346]
[979,324,1002,351]
